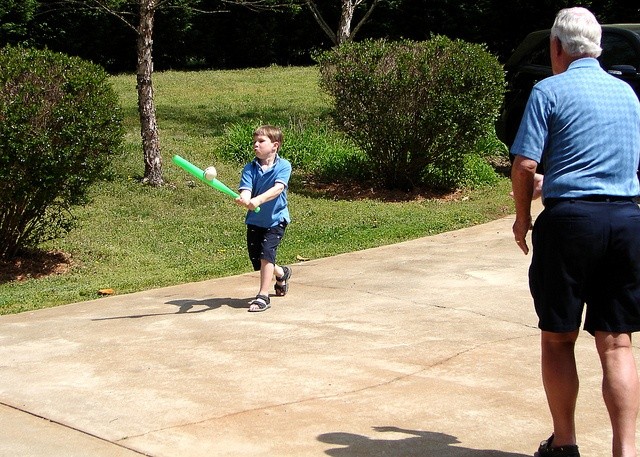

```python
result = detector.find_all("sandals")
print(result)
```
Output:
[275,266,292,296]
[249,295,271,311]
[535,433,580,456]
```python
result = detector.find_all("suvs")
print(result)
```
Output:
[504,23,640,173]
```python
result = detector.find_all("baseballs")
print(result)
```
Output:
[203,166,216,180]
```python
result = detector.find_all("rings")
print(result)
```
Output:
[516,240,521,242]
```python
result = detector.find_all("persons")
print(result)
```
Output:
[234,125,292,312]
[512,6,640,456]
